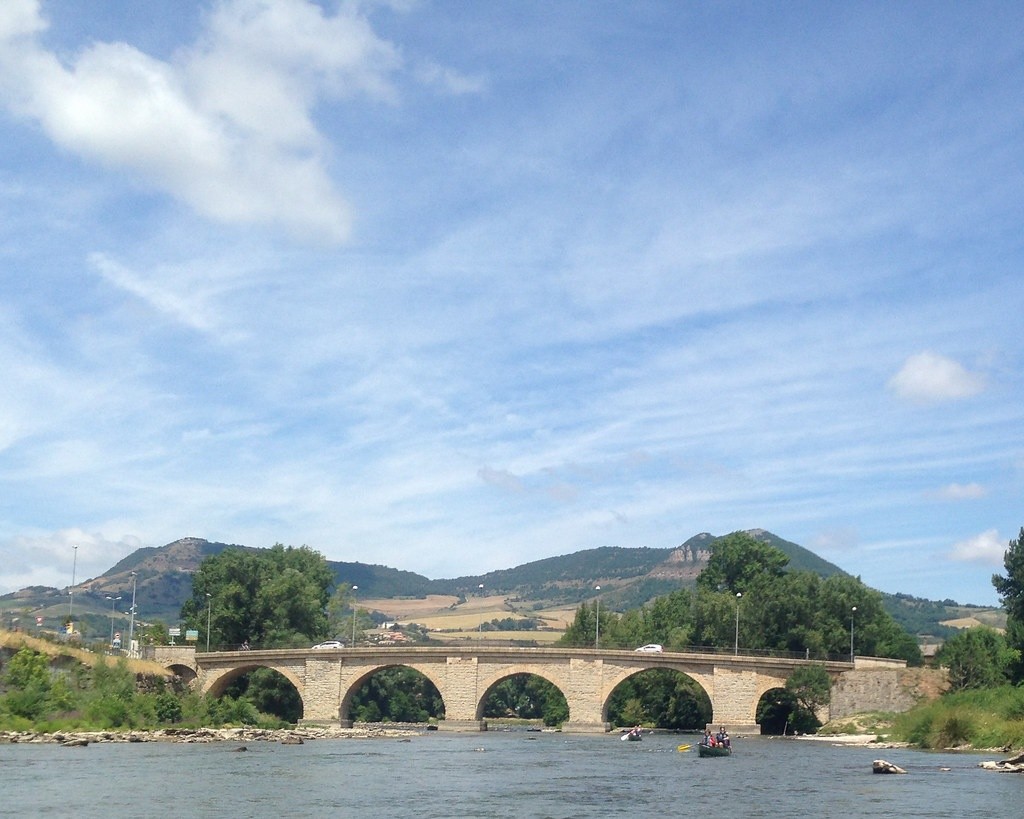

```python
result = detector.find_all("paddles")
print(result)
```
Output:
[678,740,709,750]
[621,725,641,740]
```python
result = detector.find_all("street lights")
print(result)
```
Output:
[479,584,484,647]
[125,571,138,640]
[106,595,122,645]
[595,585,601,649]
[352,586,359,648]
[205,592,212,653]
[735,592,742,655]
[851,606,857,662]
[69,545,78,615]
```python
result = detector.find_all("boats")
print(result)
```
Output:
[698,745,732,757]
[627,734,642,741]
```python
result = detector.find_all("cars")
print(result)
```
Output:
[374,631,416,645]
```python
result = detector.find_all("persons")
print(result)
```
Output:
[696,727,732,750]
[630,725,644,738]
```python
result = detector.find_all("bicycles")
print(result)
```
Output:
[238,644,250,651]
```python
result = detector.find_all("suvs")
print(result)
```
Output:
[634,644,663,653]
[311,640,345,649]
